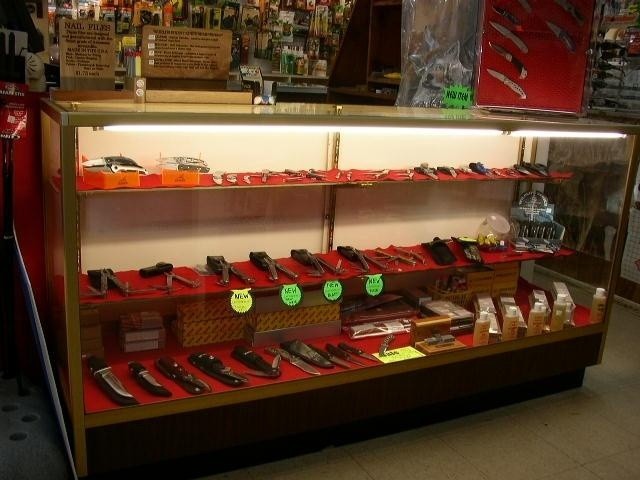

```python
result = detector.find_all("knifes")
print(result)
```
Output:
[88,357,134,399]
[489,41,528,78]
[487,69,526,100]
[547,22,576,51]
[264,334,396,375]
[421,166,457,179]
[514,164,533,176]
[492,6,521,24]
[489,21,529,53]
[128,361,163,388]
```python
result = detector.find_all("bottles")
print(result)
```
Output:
[500,306,520,342]
[527,302,545,337]
[472,310,491,347]
[590,287,608,324]
[477,212,510,252]
[126,50,142,77]
[550,293,567,332]
[295,46,305,75]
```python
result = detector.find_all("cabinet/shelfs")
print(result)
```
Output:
[40,96,640,480]
